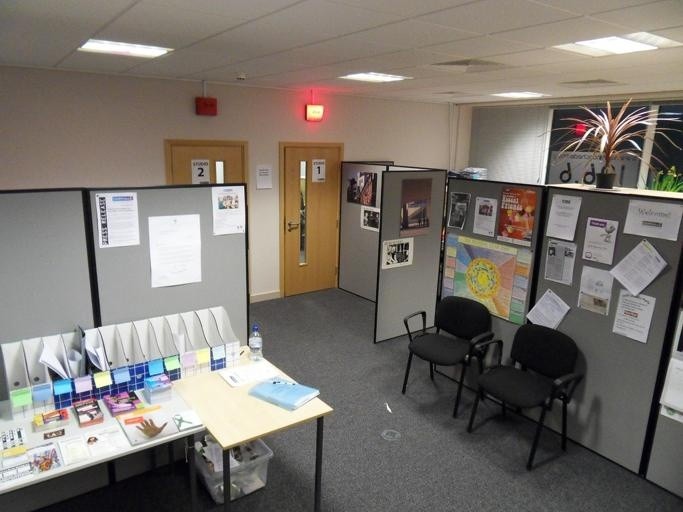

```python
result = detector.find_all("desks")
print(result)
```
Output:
[0,344,332,512]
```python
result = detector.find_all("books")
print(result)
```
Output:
[247,374,321,412]
[0,373,204,482]
[217,360,273,388]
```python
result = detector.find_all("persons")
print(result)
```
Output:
[348,179,360,203]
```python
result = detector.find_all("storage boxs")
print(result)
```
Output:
[187,433,274,506]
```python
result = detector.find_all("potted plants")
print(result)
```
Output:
[537,97,682,189]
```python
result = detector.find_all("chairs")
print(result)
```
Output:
[399,295,586,472]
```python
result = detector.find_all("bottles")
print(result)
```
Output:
[247,324,263,363]
